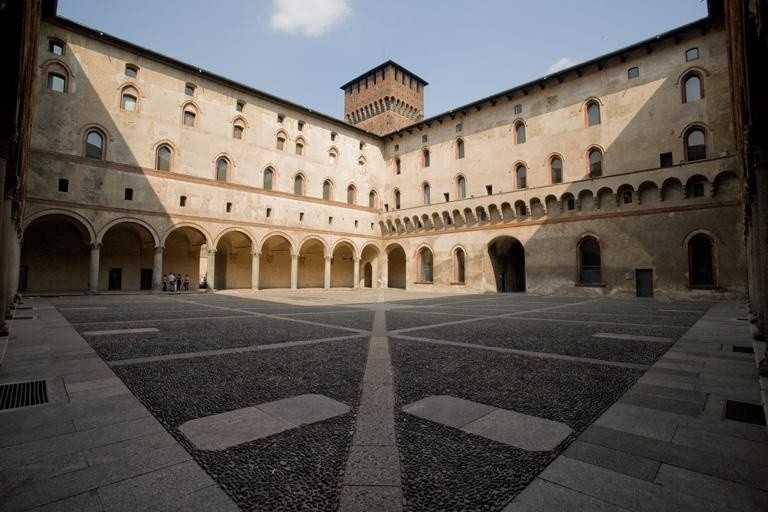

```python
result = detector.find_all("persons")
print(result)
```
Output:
[163,273,189,292]
